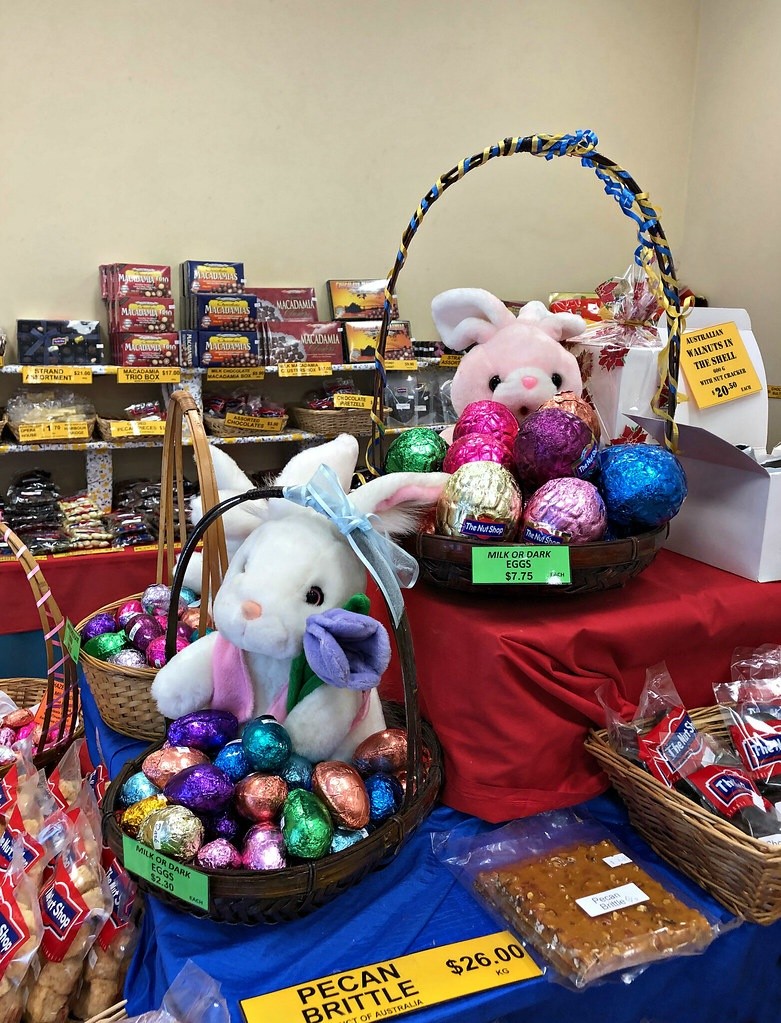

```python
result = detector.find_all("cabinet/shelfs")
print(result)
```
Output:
[0,363,379,455]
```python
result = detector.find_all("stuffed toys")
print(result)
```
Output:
[150,431,451,761]
[431,289,587,446]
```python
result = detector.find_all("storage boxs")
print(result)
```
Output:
[17,260,415,363]
[622,305,781,583]
[362,544,781,824]
[567,339,665,442]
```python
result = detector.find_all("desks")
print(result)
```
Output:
[78,676,781,1023]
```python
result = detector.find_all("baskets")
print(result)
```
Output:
[0,408,8,438]
[73,389,233,744]
[582,702,781,927]
[96,414,164,443]
[0,523,85,781]
[101,488,443,927]
[7,416,96,445]
[352,136,670,600]
[291,405,393,440]
[202,411,289,437]
